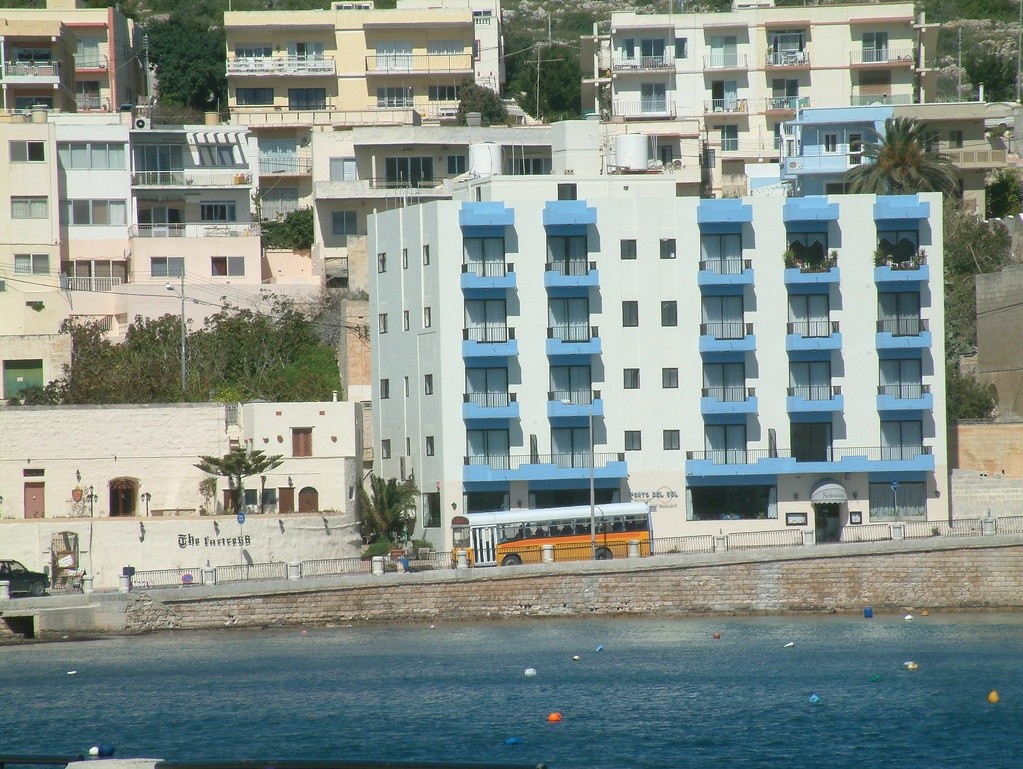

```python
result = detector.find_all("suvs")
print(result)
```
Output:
[0,559,51,598]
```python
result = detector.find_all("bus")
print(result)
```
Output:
[449,501,653,568]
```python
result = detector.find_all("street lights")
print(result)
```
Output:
[87,484,99,576]
[261,476,266,513]
[141,491,152,518]
[561,398,596,561]
[167,284,187,389]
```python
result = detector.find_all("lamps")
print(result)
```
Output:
[76,470,80,479]
[451,503,457,510]
[794,492,799,500]
[288,476,293,487]
[935,491,941,499]
[853,492,857,499]
[517,500,522,508]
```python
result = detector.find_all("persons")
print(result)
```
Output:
[519,517,635,540]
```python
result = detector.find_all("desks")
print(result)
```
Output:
[205,227,230,236]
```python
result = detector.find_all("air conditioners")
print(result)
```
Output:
[135,117,152,130]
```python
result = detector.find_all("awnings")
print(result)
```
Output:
[811,478,847,504]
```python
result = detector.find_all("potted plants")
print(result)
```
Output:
[873,245,927,270]
[391,542,405,560]
[785,248,837,273]
